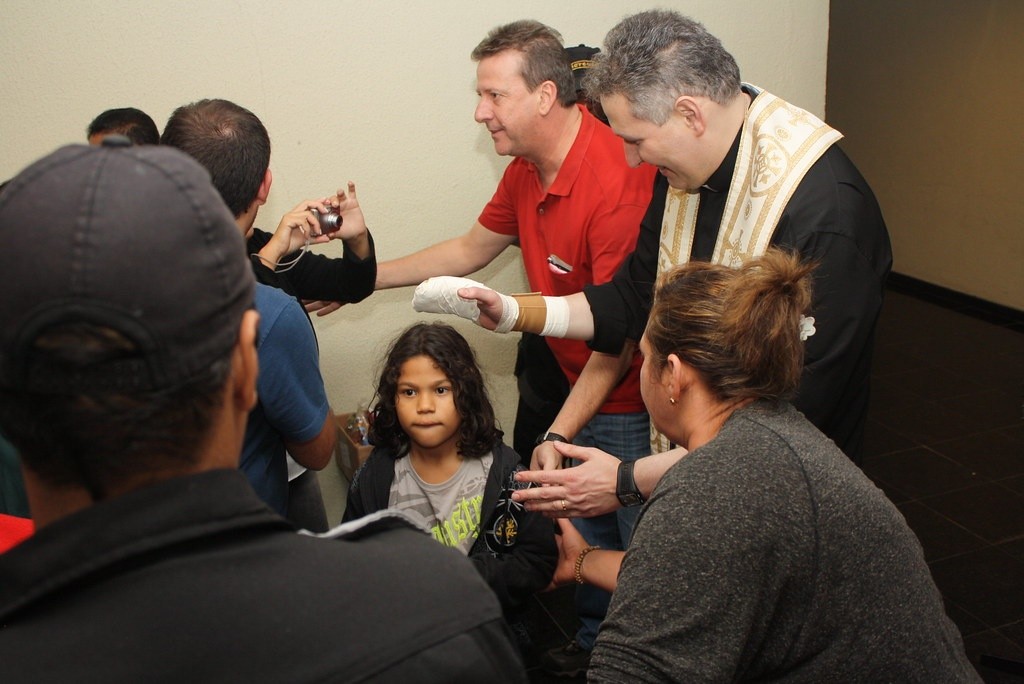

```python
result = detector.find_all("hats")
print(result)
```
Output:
[562,44,602,93]
[0,135,256,397]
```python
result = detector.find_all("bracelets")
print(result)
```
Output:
[536,432,569,445]
[574,546,601,584]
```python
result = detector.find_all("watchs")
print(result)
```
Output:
[615,457,644,507]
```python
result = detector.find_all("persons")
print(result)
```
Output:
[340,322,563,616]
[1,9,979,683]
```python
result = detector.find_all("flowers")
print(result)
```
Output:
[798,315,817,340]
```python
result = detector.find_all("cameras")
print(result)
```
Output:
[311,204,343,237]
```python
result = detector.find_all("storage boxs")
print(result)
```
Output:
[336,411,375,482]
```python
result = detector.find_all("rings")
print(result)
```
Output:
[561,500,567,510]
